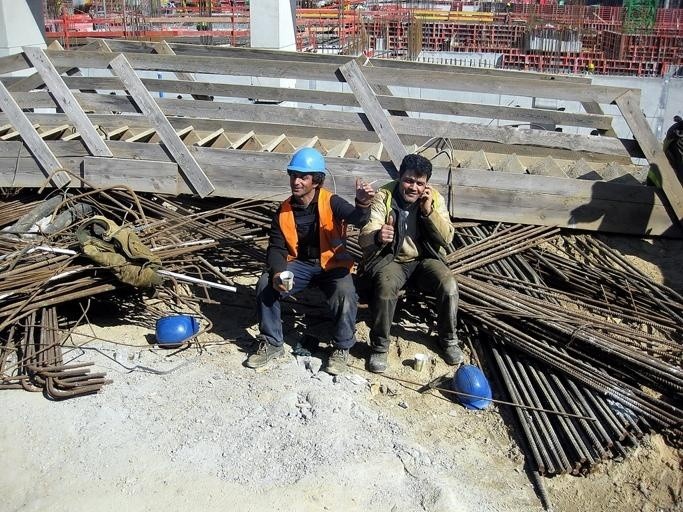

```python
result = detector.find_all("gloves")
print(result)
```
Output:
[295,335,319,356]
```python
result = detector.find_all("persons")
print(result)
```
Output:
[358,154,465,374]
[245,147,375,375]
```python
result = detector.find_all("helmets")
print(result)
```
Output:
[155,315,200,346]
[454,364,493,411]
[287,148,326,175]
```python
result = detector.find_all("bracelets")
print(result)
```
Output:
[355,202,371,208]
[421,207,433,217]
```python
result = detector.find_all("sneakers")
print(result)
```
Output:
[246,341,285,368]
[326,347,349,375]
[369,351,388,373]
[443,344,464,365]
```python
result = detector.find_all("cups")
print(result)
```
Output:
[279,270,294,292]
[413,354,428,372]
